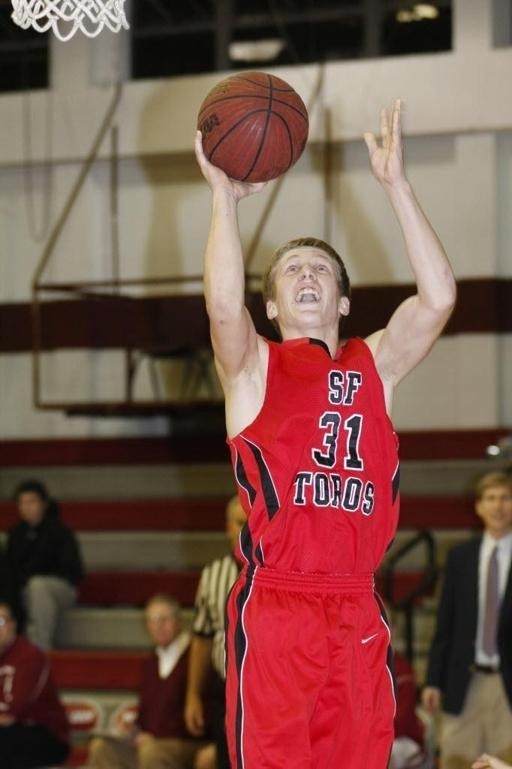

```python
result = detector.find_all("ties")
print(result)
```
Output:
[483,547,500,655]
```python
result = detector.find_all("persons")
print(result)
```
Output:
[183,491,248,768]
[420,472,512,766]
[3,478,86,653]
[86,592,207,769]
[389,654,427,766]
[1,591,72,769]
[191,99,459,768]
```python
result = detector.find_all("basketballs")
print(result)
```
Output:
[198,70,310,185]
